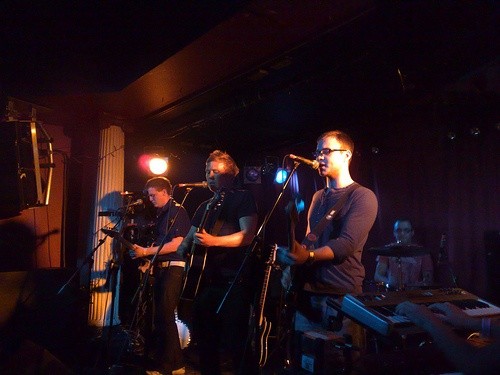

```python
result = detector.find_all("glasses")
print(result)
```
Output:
[312,148,346,158]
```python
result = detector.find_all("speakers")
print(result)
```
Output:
[0,340,75,375]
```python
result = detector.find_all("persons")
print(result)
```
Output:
[395,301,500,375]
[286,131,378,375]
[129,176,190,375]
[176,150,258,375]
[374,218,434,290]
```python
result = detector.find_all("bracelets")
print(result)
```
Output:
[143,248,147,255]
[309,251,315,260]
[480,317,491,334]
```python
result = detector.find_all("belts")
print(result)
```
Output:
[158,260,186,268]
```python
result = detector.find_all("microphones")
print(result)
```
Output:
[124,199,143,208]
[289,154,319,170]
[440,232,446,251]
[178,181,208,188]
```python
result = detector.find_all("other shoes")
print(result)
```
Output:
[171,366,185,375]
[145,370,163,375]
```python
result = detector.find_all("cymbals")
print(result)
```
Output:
[367,242,429,257]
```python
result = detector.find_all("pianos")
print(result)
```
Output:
[325,287,499,337]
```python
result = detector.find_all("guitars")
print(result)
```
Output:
[254,243,278,366]
[101,227,153,274]
[180,187,225,302]
[287,198,307,311]
